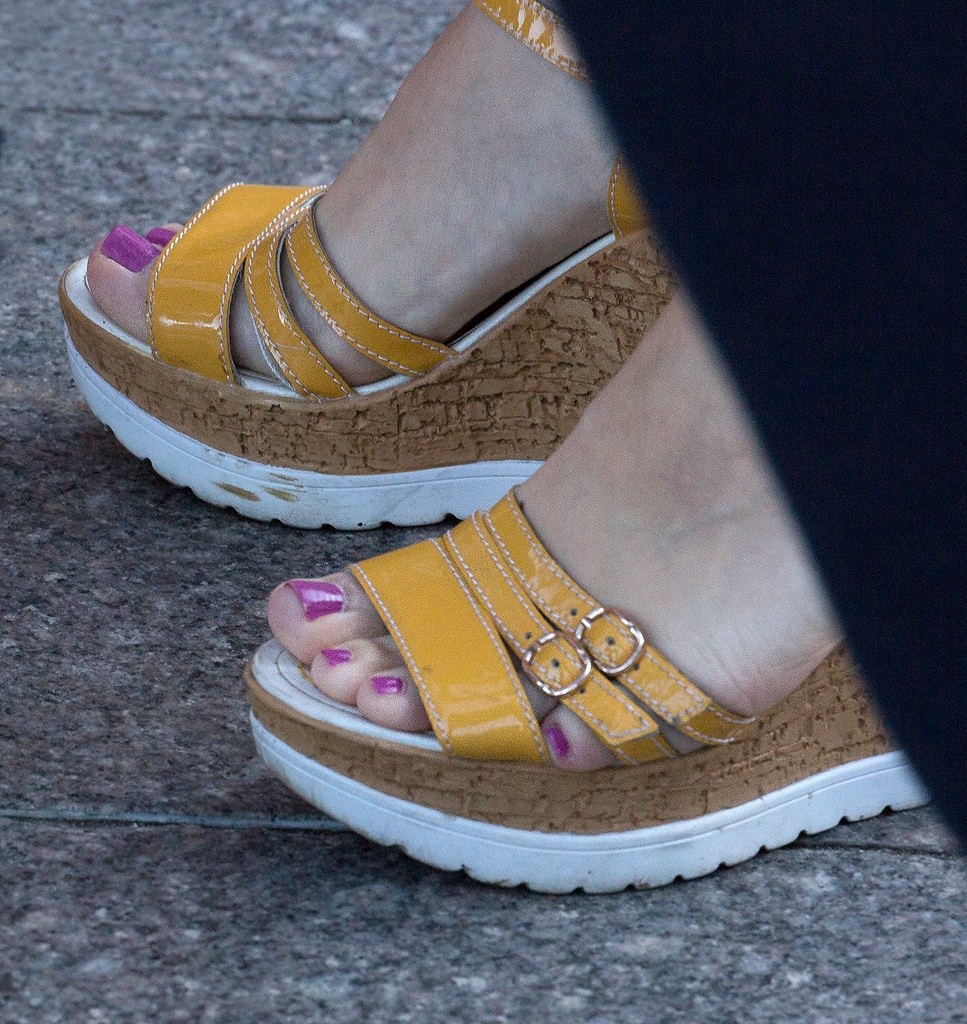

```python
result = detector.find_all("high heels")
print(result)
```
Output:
[59,0,680,532]
[244,484,931,898]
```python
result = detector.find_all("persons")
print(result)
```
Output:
[54,0,937,893]
